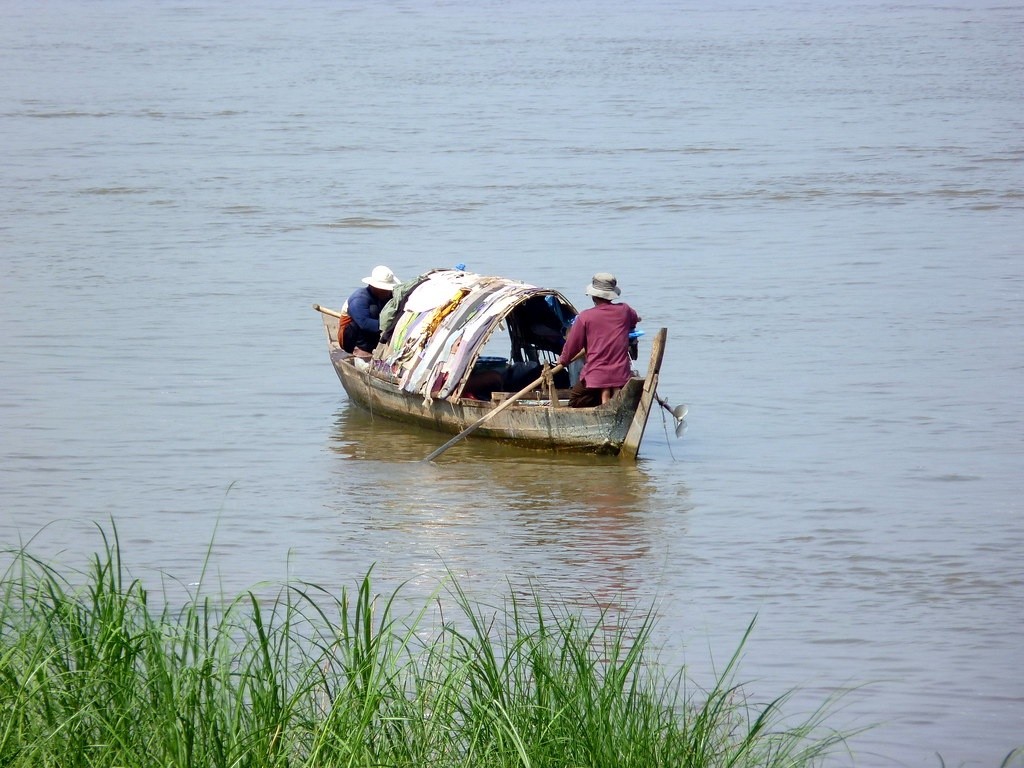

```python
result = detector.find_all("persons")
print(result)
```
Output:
[338,266,402,358]
[556,273,638,408]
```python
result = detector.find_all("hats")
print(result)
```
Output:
[361,265,400,290]
[586,272,621,300]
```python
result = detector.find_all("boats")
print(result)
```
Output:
[315,303,667,462]
